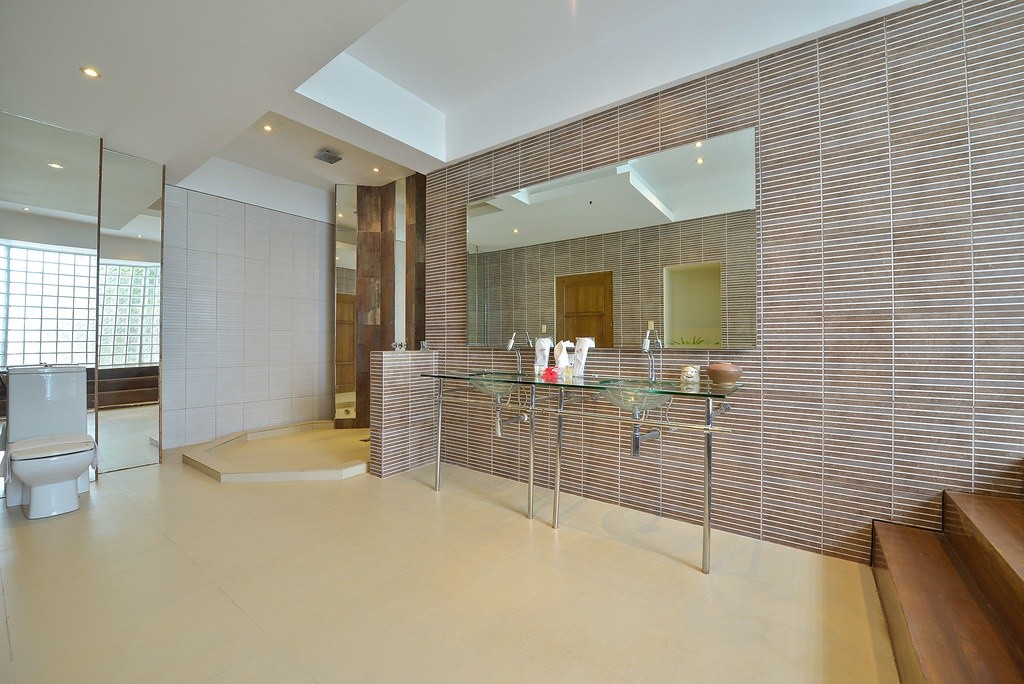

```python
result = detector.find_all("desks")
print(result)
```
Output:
[420,369,743,574]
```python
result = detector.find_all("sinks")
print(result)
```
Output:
[599,377,679,415]
[469,371,530,400]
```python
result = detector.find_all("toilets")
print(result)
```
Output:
[3,362,98,521]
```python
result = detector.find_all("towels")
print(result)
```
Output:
[4,431,104,485]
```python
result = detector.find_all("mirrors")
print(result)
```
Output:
[1,111,164,498]
[466,127,759,351]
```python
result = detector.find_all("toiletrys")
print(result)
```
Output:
[533,363,545,378]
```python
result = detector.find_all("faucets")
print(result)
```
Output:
[641,330,658,382]
[505,331,526,374]
